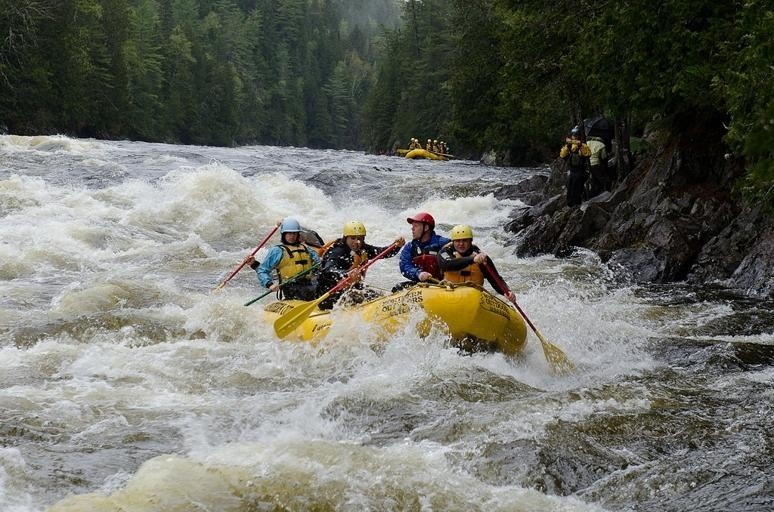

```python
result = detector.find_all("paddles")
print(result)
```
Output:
[213,222,281,292]
[482,260,577,375]
[430,151,454,158]
[274,241,399,339]
[396,149,410,154]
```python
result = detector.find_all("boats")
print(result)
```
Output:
[264,279,528,360]
[405,149,450,162]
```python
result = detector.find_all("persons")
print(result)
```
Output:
[408,137,449,156]
[560,125,591,211]
[391,213,451,294]
[256,218,322,302]
[437,225,516,304]
[586,127,609,198]
[313,220,406,311]
[241,219,324,270]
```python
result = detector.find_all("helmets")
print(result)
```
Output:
[450,224,473,241]
[343,221,366,237]
[410,138,418,143]
[571,128,580,136]
[280,218,302,234]
[407,213,435,226]
[428,139,446,144]
